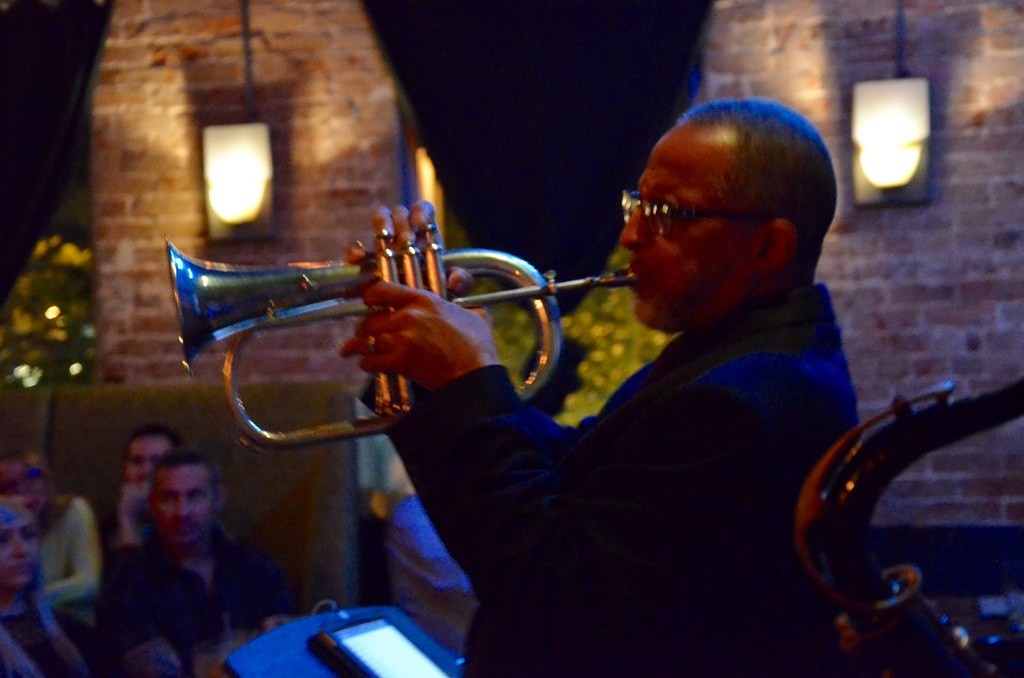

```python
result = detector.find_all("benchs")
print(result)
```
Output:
[1,377,357,629]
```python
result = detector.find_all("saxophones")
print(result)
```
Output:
[790,374,1024,678]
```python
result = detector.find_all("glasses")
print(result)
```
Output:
[621,189,789,238]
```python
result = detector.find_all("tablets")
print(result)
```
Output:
[317,615,450,678]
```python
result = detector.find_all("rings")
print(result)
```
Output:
[367,336,376,351]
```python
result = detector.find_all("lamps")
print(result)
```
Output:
[200,0,279,243]
[852,0,936,207]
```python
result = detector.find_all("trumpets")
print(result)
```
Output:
[162,219,638,451]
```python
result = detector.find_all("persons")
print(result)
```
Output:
[341,98,860,678]
[1,427,287,678]
